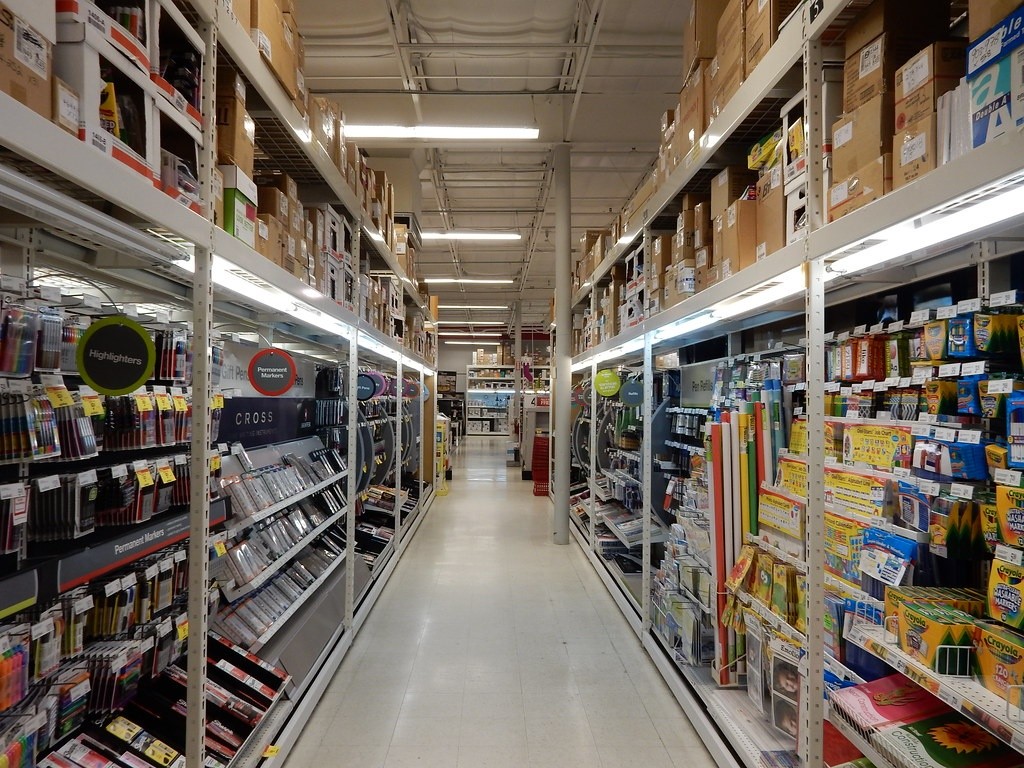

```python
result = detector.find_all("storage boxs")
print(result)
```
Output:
[549,0,1024,359]
[0,0,438,371]
[467,407,507,432]
[469,344,550,390]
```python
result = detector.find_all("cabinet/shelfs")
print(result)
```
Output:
[463,365,552,435]
[826,601,1024,768]
[520,405,550,481]
[577,449,675,550]
[211,432,373,701]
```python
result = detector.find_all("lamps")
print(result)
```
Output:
[420,210,522,241]
[343,95,540,140]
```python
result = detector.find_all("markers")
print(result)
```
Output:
[0,550,222,768]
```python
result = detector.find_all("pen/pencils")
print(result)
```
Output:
[1,303,225,566]
